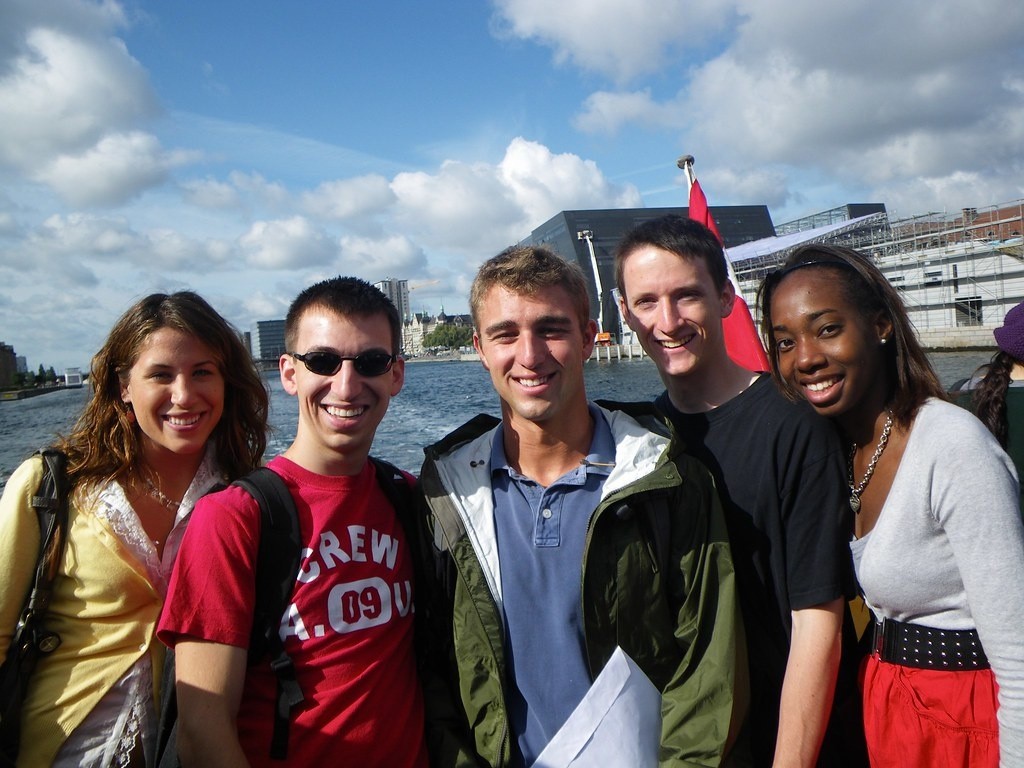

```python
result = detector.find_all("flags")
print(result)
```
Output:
[686,179,773,375]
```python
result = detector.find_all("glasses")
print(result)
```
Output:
[281,350,403,376]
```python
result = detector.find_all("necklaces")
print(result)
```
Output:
[131,461,181,512]
[846,404,895,514]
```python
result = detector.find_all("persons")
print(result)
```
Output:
[156,272,435,768]
[408,245,750,767]
[753,238,1024,768]
[0,288,278,768]
[613,213,856,768]
[948,301,1024,492]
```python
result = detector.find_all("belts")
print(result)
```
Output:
[865,618,991,670]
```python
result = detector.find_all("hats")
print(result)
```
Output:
[993,299,1024,361]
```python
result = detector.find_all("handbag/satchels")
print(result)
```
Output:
[0,448,68,768]
